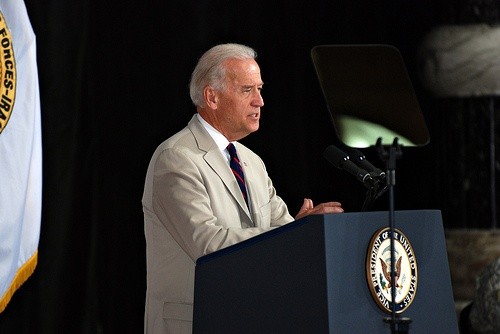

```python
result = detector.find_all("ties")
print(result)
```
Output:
[227,143,249,210]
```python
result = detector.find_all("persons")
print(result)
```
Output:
[140,44,344,334]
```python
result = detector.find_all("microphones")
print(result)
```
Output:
[323,145,386,189]
